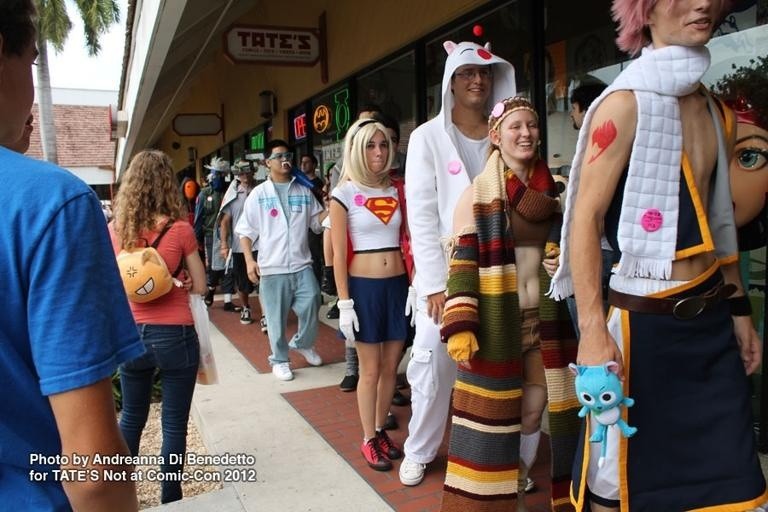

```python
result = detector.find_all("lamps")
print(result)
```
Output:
[258,88,278,118]
[186,146,197,162]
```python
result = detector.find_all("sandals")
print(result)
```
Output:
[223,301,242,312]
[205,290,214,307]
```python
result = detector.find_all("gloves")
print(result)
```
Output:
[446,330,480,363]
[336,298,361,345]
[405,284,420,329]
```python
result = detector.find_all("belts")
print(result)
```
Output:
[607,277,738,317]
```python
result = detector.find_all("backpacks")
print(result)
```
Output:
[116,218,184,304]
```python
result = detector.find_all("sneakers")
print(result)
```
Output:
[524,478,535,492]
[385,410,397,429]
[327,298,340,319]
[360,429,402,472]
[291,346,323,366]
[272,361,293,381]
[398,453,427,487]
[392,391,408,408]
[260,316,267,332]
[339,374,358,392]
[239,306,252,325]
[396,375,408,390]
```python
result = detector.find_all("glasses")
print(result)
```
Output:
[453,68,491,79]
[267,152,295,160]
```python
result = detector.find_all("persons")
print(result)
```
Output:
[179,0,768,510]
[100,202,113,224]
[1,0,139,512]
[109,150,209,502]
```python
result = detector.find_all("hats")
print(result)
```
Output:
[487,96,540,131]
